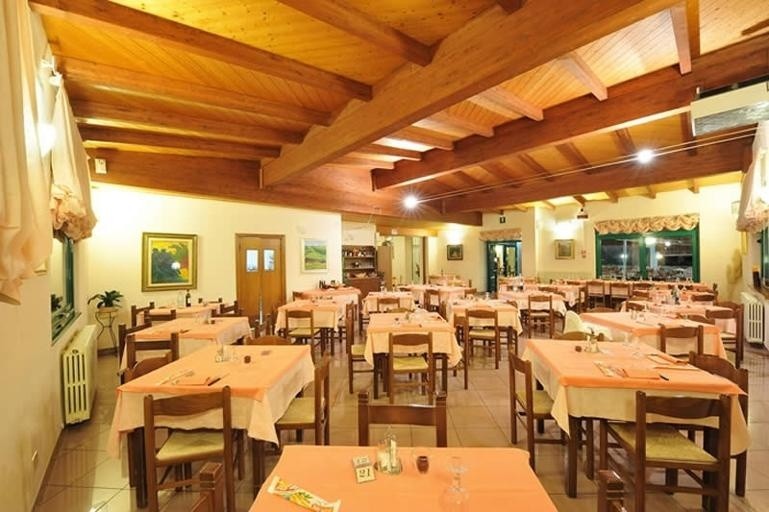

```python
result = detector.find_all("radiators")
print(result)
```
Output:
[64,323,99,426]
[738,292,762,343]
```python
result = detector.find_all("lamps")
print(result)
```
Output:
[576,203,589,220]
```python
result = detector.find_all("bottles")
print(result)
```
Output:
[186,289,190,307]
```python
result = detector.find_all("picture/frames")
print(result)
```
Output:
[552,239,573,259]
[447,245,462,260]
[142,232,199,291]
[301,239,328,274]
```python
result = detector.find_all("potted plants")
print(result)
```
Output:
[87,290,125,317]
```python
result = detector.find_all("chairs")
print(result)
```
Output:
[386,306,418,314]
[449,313,469,390]
[524,293,555,339]
[210,308,242,319]
[465,307,500,369]
[244,336,290,348]
[291,290,302,299]
[502,298,519,352]
[586,280,606,306]
[337,300,353,343]
[253,313,272,337]
[465,288,478,298]
[596,386,733,512]
[198,296,223,306]
[252,351,331,502]
[631,280,654,290]
[426,289,440,310]
[609,282,631,307]
[357,293,369,333]
[220,299,237,316]
[144,386,246,512]
[346,318,386,393]
[123,351,193,506]
[357,388,448,451]
[553,330,604,341]
[507,352,593,479]
[659,324,703,358]
[591,307,614,314]
[706,308,739,369]
[142,308,177,327]
[124,332,178,365]
[680,350,749,499]
[689,283,718,295]
[117,316,155,383]
[550,292,565,299]
[386,332,433,406]
[440,301,448,322]
[348,299,355,343]
[284,309,315,364]
[630,289,650,300]
[687,314,715,325]
[688,289,718,305]
[538,284,558,292]
[575,284,581,316]
[376,297,400,314]
[184,460,226,512]
[130,302,155,328]
[624,300,646,313]
[714,300,745,361]
[399,287,411,292]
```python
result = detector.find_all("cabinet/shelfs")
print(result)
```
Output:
[376,245,394,292]
[341,243,380,292]
